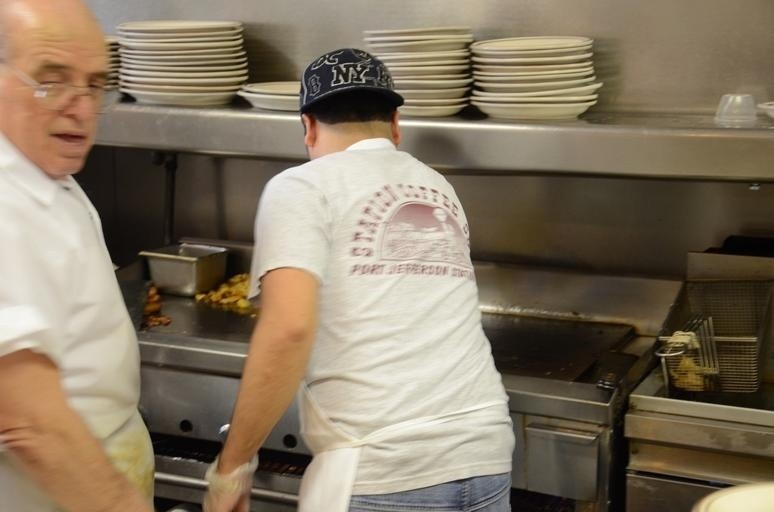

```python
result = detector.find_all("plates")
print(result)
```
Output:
[105,22,301,112]
[363,27,604,120]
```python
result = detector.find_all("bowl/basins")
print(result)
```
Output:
[713,92,759,123]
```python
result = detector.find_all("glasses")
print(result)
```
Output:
[21,72,122,114]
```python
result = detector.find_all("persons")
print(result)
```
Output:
[199,47,517,511]
[0,0,158,512]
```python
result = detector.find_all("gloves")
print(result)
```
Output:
[201,451,261,511]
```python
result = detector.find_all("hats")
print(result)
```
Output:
[298,46,403,113]
